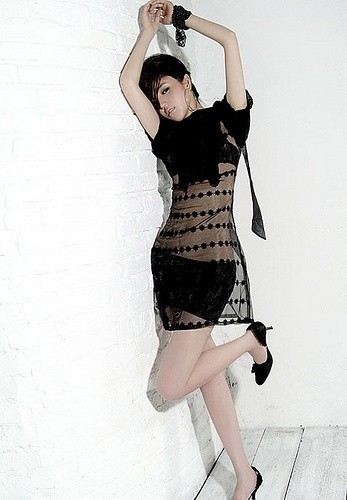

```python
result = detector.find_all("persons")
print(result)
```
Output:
[119,0,273,500]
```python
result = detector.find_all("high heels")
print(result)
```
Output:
[247,466,263,500]
[246,322,273,385]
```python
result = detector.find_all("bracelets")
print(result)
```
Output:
[170,4,191,47]
